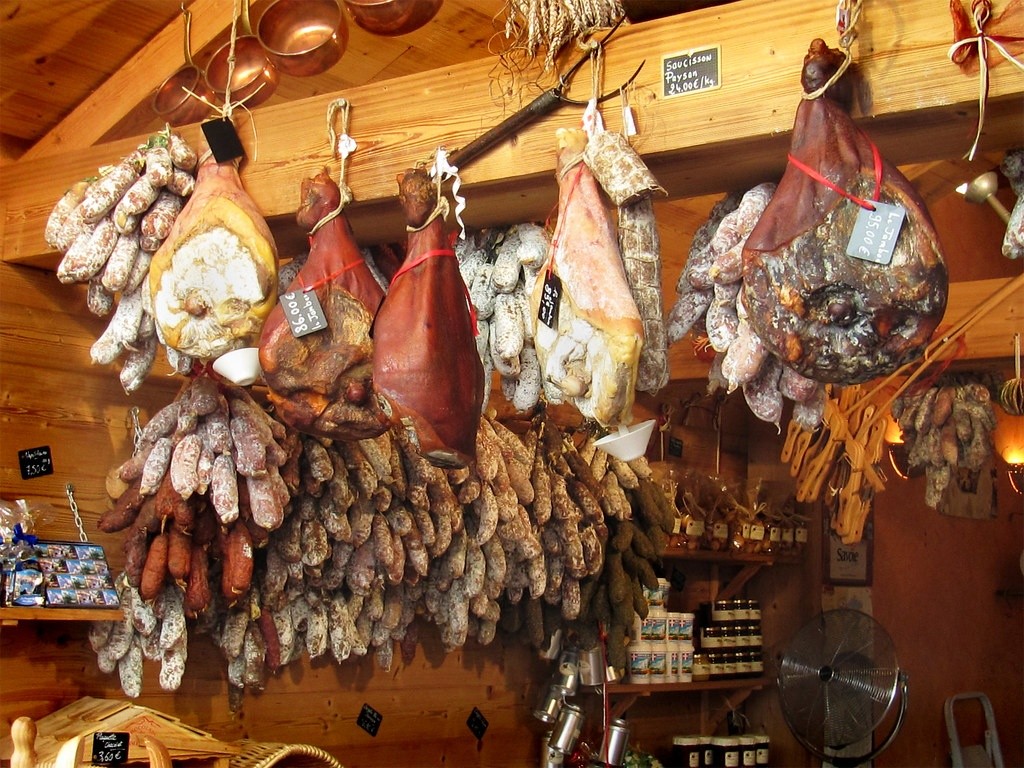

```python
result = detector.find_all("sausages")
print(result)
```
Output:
[891,145,1021,513]
[87,373,675,716]
[583,133,826,431]
[450,221,567,409]
[43,123,198,387]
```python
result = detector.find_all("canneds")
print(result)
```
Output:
[670,733,770,768]
[694,597,765,680]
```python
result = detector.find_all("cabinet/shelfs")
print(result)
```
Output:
[568,454,818,768]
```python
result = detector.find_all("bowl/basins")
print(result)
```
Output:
[593,419,657,463]
[213,348,261,386]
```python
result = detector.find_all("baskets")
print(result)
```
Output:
[228,738,346,768]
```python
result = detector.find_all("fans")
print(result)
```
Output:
[774,606,909,768]
